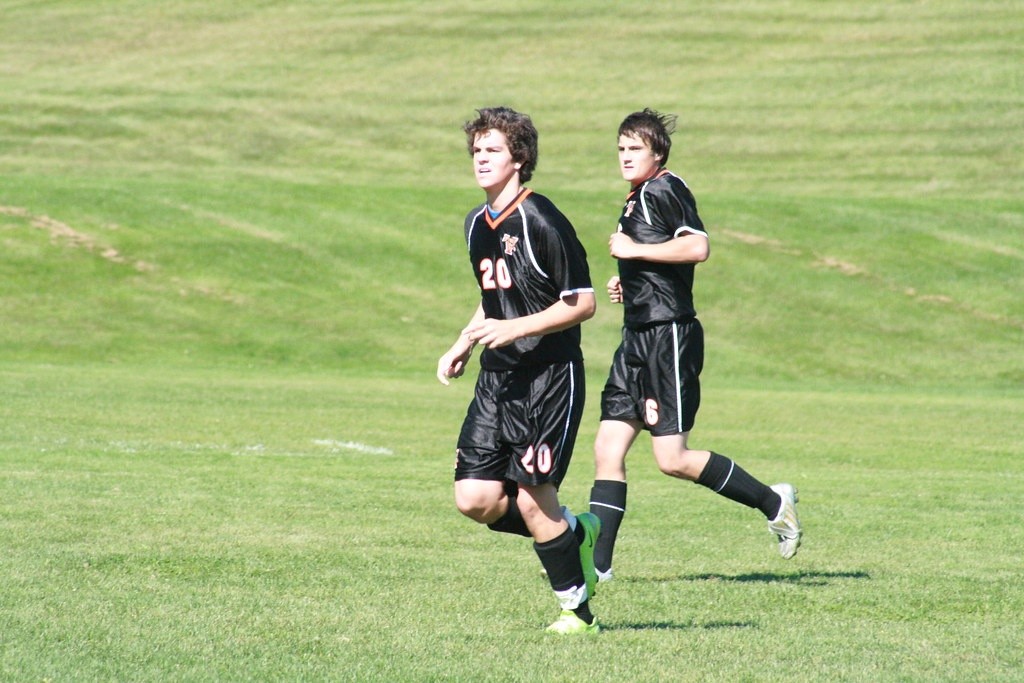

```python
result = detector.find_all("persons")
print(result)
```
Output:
[436,107,603,636]
[542,104,804,578]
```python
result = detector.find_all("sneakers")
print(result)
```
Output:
[544,610,601,641]
[575,512,601,601]
[768,483,803,560]
[539,567,614,582]
